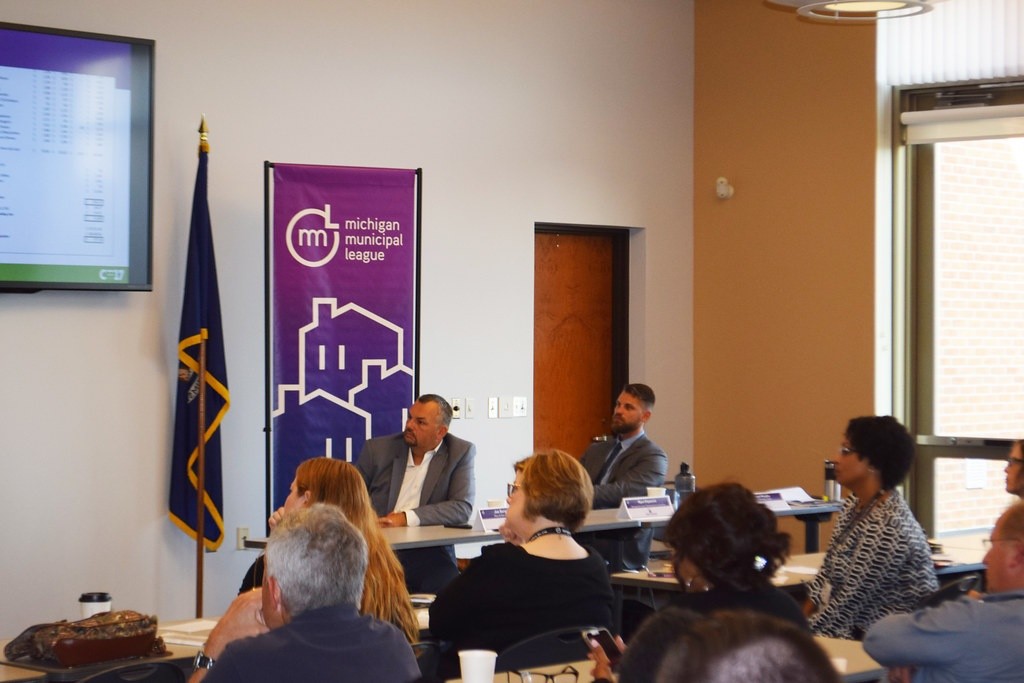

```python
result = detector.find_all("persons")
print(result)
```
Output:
[578,383,669,576]
[355,394,477,597]
[587,482,812,683]
[416,448,616,677]
[188,501,422,683]
[655,607,841,683]
[237,456,426,659]
[617,605,709,683]
[1004,440,1024,500]
[862,500,1024,683]
[802,416,941,641]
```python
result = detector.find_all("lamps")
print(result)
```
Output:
[766,0,951,24]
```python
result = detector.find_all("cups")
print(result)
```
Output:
[77,592,113,619]
[646,486,666,497]
[487,499,507,509]
[458,650,497,683]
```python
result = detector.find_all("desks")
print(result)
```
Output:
[244,513,640,574]
[611,552,826,636]
[445,634,888,683]
[0,607,431,683]
[590,498,843,554]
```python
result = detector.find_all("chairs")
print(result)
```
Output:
[76,481,989,683]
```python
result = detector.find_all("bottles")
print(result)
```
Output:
[825,460,842,502]
[674,462,697,510]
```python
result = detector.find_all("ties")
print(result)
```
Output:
[592,443,621,487]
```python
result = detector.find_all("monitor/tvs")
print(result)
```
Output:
[0,22,156,293]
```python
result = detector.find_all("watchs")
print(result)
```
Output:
[191,651,216,672]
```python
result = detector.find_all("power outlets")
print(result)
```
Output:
[237,527,249,550]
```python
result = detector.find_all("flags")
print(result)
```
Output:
[168,142,232,551]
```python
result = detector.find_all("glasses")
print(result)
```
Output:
[842,447,858,456]
[508,483,520,498]
[1007,458,1024,466]
[983,538,1020,551]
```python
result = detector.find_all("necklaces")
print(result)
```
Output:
[835,489,886,543]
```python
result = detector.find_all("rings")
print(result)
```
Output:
[250,587,258,593]
[387,519,392,523]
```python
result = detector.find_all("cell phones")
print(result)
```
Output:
[582,626,625,676]
